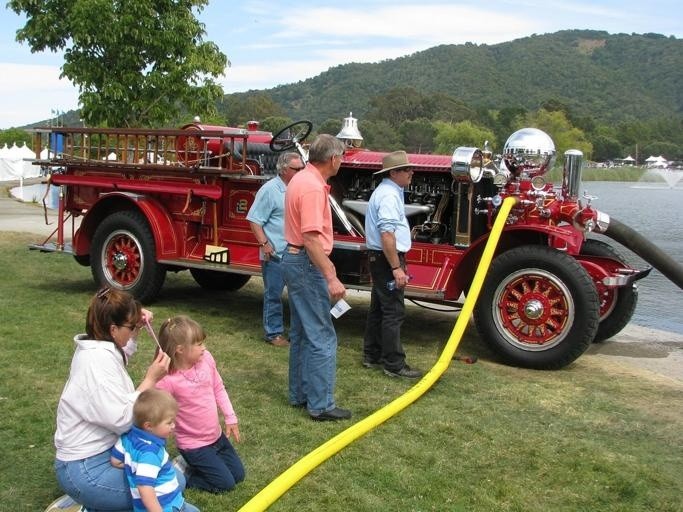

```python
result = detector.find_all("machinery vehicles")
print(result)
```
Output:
[21,110,682,370]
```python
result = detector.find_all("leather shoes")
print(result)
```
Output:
[383,365,422,379]
[290,402,307,409]
[264,334,290,346]
[362,360,383,368]
[310,407,351,422]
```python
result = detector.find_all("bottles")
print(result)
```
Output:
[387,274,413,290]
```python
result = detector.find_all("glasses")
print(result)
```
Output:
[289,166,304,171]
[114,323,136,330]
[395,166,410,172]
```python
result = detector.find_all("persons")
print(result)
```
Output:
[364,151,423,380]
[150,315,245,496]
[280,133,351,420]
[244,151,304,348]
[111,388,201,512]
[42,287,169,512]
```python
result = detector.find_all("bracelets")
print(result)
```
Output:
[257,240,268,248]
[391,264,401,271]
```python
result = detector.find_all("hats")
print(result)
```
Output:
[372,150,418,175]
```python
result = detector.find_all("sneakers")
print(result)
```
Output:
[171,454,187,473]
[44,493,88,512]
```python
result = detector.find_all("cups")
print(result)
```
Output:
[330,298,351,320]
